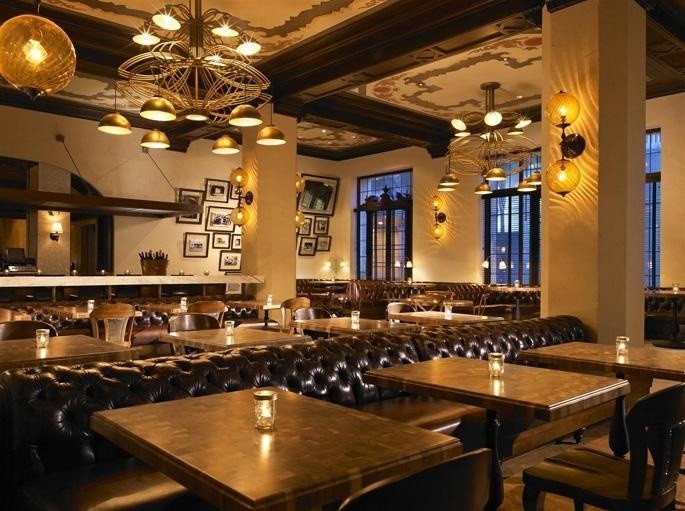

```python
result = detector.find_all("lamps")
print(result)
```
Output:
[498,254,506,270]
[0,4,76,101]
[49,222,63,241]
[435,81,543,194]
[405,256,412,268]
[546,88,586,198]
[482,255,494,269]
[296,173,306,227]
[394,258,405,267]
[98,0,287,154]
[429,196,446,239]
[230,166,254,228]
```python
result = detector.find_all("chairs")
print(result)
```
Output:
[1,321,58,341]
[292,306,332,334]
[167,314,224,356]
[337,449,493,511]
[280,297,310,332]
[387,301,415,321]
[90,304,135,348]
[187,301,226,328]
[523,383,685,511]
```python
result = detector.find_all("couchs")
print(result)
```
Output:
[486,286,541,307]
[345,280,410,315]
[645,287,684,326]
[0,326,490,509]
[412,315,588,365]
[436,282,485,313]
[296,279,351,297]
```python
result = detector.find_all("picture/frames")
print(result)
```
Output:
[212,232,231,249]
[231,185,242,200]
[298,218,312,236]
[204,206,236,233]
[316,236,331,251]
[183,232,210,258]
[313,216,329,235]
[219,250,241,271]
[205,178,230,203]
[232,234,241,251]
[298,236,317,256]
[176,189,205,225]
[225,272,244,295]
[296,173,340,216]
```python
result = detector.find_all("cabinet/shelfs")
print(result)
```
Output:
[0,275,265,301]
